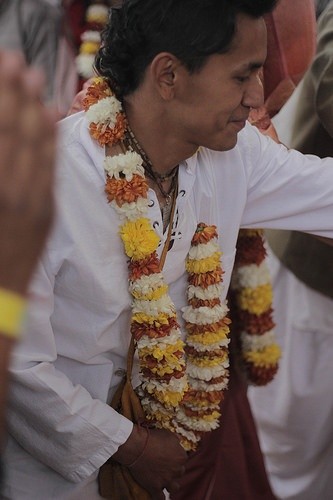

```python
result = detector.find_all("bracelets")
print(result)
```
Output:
[0,287,34,340]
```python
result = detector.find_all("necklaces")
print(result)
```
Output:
[79,75,245,450]
[121,106,183,235]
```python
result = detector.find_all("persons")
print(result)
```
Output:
[5,0,333,500]
[0,49,58,457]
[238,0,333,499]
